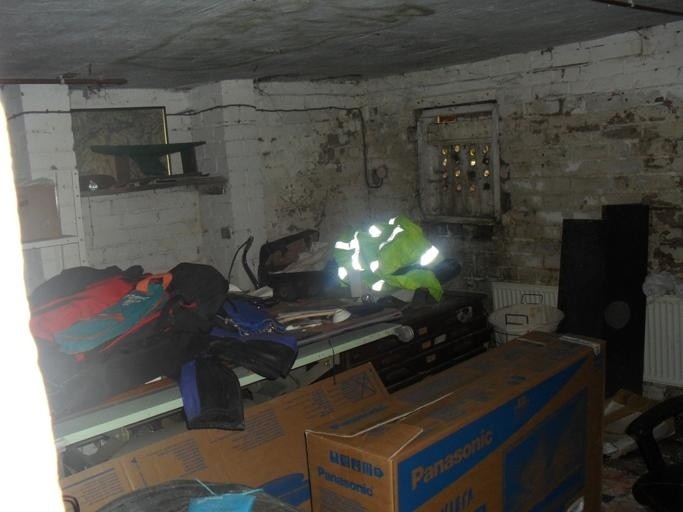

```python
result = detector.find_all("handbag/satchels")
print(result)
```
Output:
[29,272,183,365]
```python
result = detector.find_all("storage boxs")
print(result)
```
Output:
[59,360,389,511]
[16,183,62,242]
[307,330,607,511]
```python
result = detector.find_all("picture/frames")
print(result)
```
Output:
[70,105,172,196]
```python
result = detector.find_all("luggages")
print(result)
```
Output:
[259,229,336,302]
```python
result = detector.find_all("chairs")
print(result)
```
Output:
[625,395,683,512]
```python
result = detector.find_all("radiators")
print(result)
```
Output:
[492,282,682,391]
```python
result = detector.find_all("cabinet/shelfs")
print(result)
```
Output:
[341,291,493,393]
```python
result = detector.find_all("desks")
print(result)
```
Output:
[53,321,404,480]
